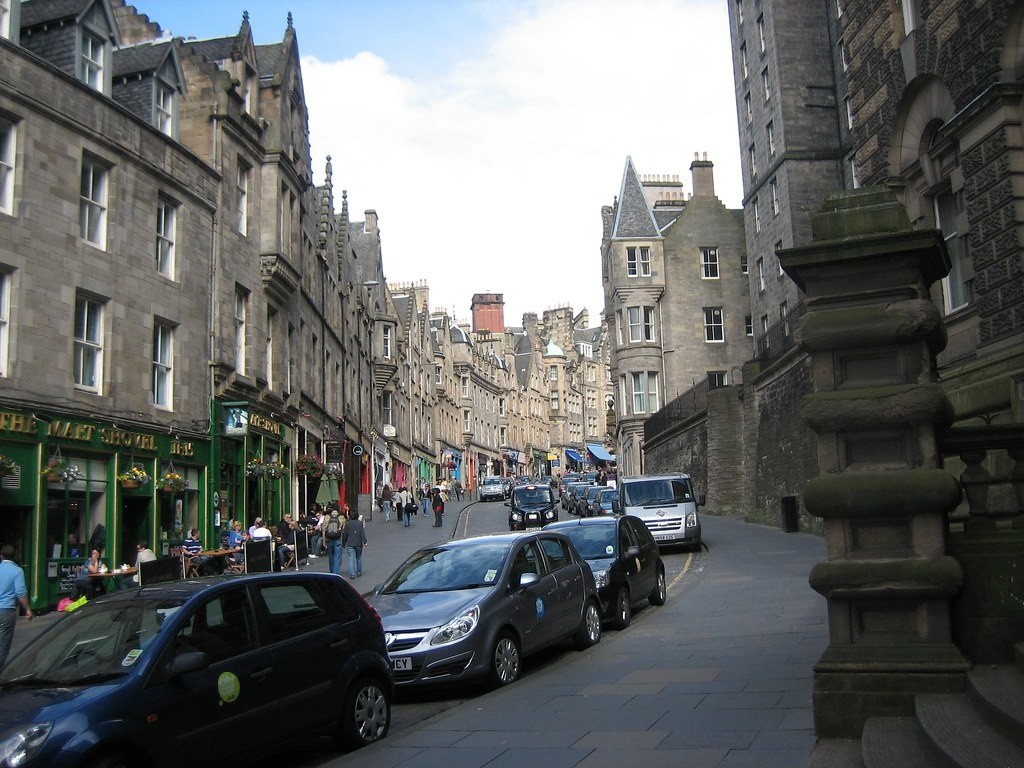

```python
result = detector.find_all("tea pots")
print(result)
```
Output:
[121,563,130,572]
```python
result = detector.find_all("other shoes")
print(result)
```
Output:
[432,524,442,528]
[424,513,426,516]
[281,565,285,569]
[427,515,428,517]
[308,554,319,559]
[350,575,355,579]
[357,573,362,577]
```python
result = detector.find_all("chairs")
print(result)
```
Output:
[606,530,623,540]
[447,552,476,585]
[603,494,609,498]
[510,546,529,588]
[211,593,245,647]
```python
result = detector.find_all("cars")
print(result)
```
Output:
[363,532,601,693]
[568,485,594,514]
[578,485,613,517]
[526,515,667,631]
[502,475,540,498]
[563,474,579,480]
[586,473,597,483]
[558,478,577,495]
[561,482,590,510]
[0,569,393,768]
[593,488,618,516]
[539,476,557,488]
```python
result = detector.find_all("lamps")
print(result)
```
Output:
[349,280,379,287]
[113,422,130,431]
[235,422,242,427]
[290,421,298,427]
[32,412,53,424]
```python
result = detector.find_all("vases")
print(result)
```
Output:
[48,473,60,481]
[162,483,173,492]
[121,480,140,488]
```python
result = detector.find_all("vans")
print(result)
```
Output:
[613,472,705,553]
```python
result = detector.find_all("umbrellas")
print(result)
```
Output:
[315,473,341,507]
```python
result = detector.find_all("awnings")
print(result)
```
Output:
[565,449,584,462]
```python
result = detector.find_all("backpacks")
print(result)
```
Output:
[326,513,343,539]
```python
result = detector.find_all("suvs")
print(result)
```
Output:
[480,476,506,502]
[504,485,560,530]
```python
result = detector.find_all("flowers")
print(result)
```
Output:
[159,473,189,492]
[246,459,286,481]
[296,454,342,480]
[117,467,152,484]
[0,454,15,475]
[449,462,457,469]
[41,460,85,482]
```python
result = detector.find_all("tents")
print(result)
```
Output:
[587,443,616,461]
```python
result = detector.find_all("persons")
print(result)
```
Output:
[106,612,209,676]
[557,464,617,487]
[376,477,462,527]
[67,503,369,600]
[0,545,33,667]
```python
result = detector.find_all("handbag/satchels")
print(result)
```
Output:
[404,492,419,513]
[436,505,442,512]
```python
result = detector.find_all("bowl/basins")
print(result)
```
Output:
[98,568,108,574]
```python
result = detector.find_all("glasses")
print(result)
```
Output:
[289,517,292,518]
[425,486,428,487]
[137,547,142,550]
[439,482,442,483]
[316,514,320,517]
